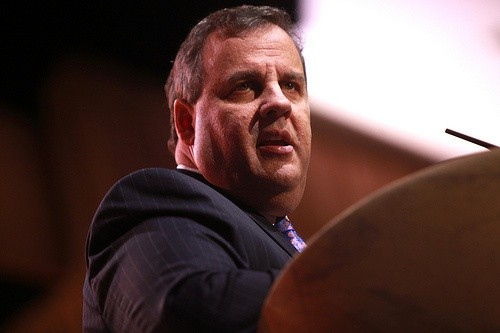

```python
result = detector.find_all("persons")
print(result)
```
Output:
[82,6,312,333]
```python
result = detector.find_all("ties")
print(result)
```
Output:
[274,217,307,252]
